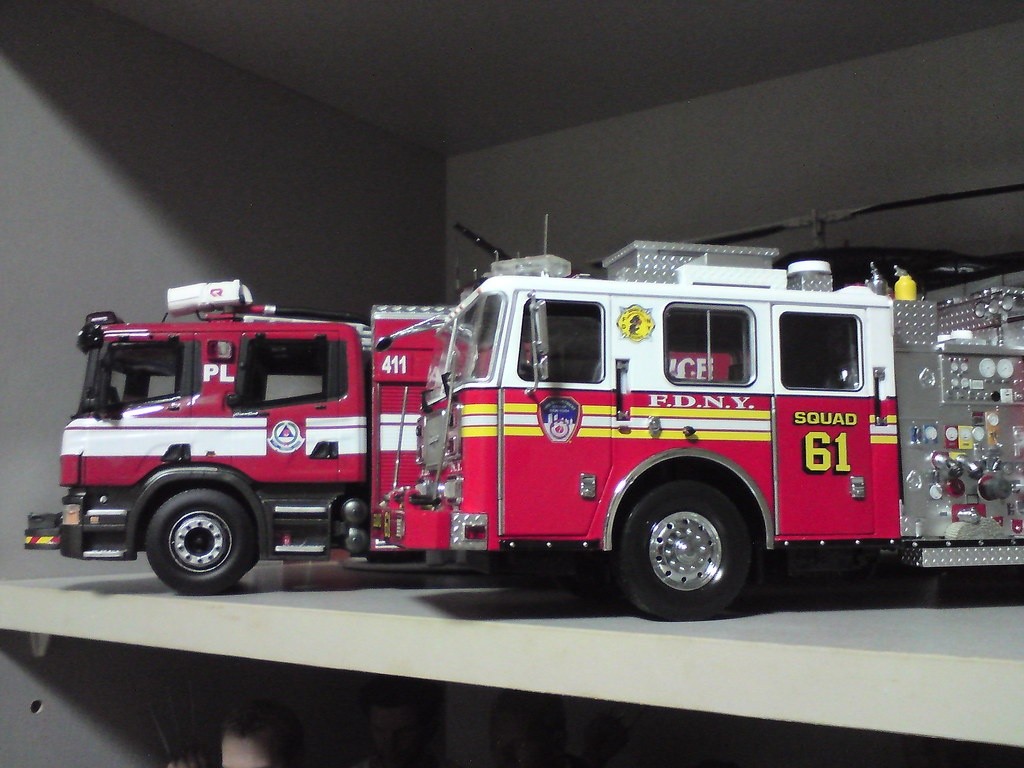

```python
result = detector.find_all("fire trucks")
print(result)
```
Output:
[19,274,731,597]
[376,209,1023,624]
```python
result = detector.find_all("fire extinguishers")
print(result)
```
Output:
[866,261,918,300]
